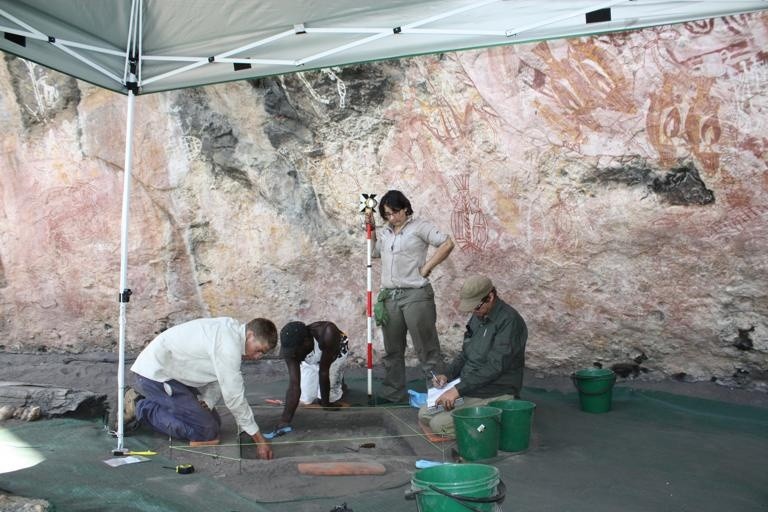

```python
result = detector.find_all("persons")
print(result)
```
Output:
[418,274,528,437]
[365,190,455,405]
[109,317,277,460]
[272,320,349,438]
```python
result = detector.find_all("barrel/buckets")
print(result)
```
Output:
[488,399,536,452]
[451,406,502,460]
[404,464,506,512]
[571,368,615,413]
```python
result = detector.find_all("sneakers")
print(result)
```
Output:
[109,386,146,432]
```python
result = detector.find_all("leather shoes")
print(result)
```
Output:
[367,396,390,405]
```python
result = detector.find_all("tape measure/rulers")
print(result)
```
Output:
[162,463,194,474]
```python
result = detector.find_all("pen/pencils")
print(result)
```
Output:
[430,371,444,387]
[123,452,159,455]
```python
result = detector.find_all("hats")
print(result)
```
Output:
[279,322,309,358]
[458,275,493,312]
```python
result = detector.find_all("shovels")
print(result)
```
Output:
[343,442,376,452]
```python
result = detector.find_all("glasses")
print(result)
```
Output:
[383,210,400,218]
[474,298,488,310]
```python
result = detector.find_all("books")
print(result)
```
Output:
[427,378,464,416]
